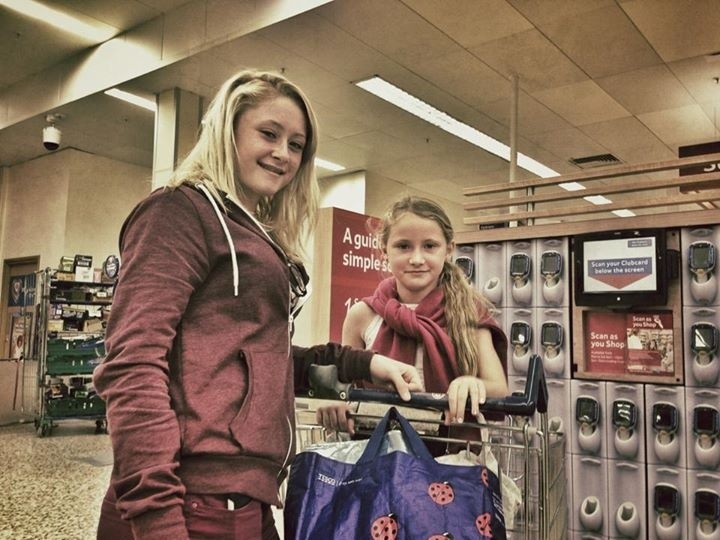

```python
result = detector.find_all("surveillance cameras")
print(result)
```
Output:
[42,126,63,151]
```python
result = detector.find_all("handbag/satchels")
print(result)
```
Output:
[285,406,505,540]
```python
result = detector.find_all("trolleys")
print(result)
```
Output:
[289,354,567,540]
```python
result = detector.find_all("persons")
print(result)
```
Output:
[13,334,23,358]
[315,195,511,459]
[92,68,425,540]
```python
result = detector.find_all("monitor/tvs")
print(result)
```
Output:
[573,228,668,307]
